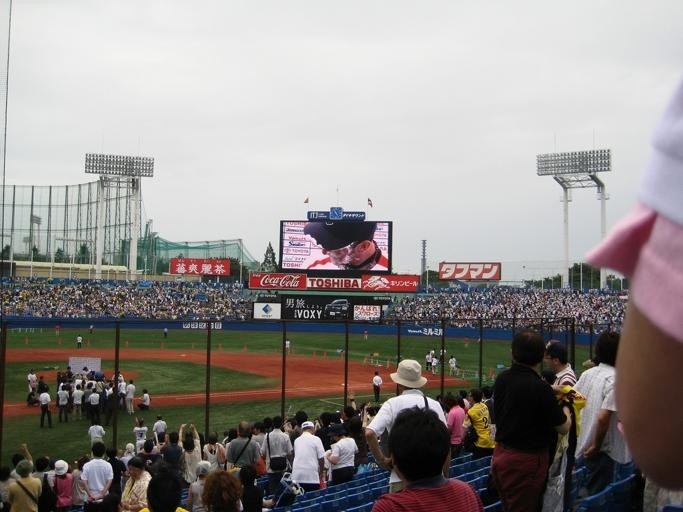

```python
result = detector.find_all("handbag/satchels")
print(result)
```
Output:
[216,443,227,465]
[464,415,478,443]
[0,495,11,512]
[273,472,305,507]
[270,456,287,470]
[256,452,266,474]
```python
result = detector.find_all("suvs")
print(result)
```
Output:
[324,299,350,319]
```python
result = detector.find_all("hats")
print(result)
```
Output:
[54,460,69,475]
[328,424,348,436]
[16,460,34,474]
[544,339,567,363]
[195,460,211,476]
[301,420,314,429]
[303,222,377,251]
[390,359,427,389]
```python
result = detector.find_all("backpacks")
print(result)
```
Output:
[39,473,55,511]
[71,474,87,503]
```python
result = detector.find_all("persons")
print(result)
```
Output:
[2,394,366,512]
[366,337,635,512]
[285,338,290,351]
[24,363,151,430]
[392,282,630,338]
[302,221,388,272]
[0,270,260,324]
[76,334,82,348]
[585,76,683,495]
